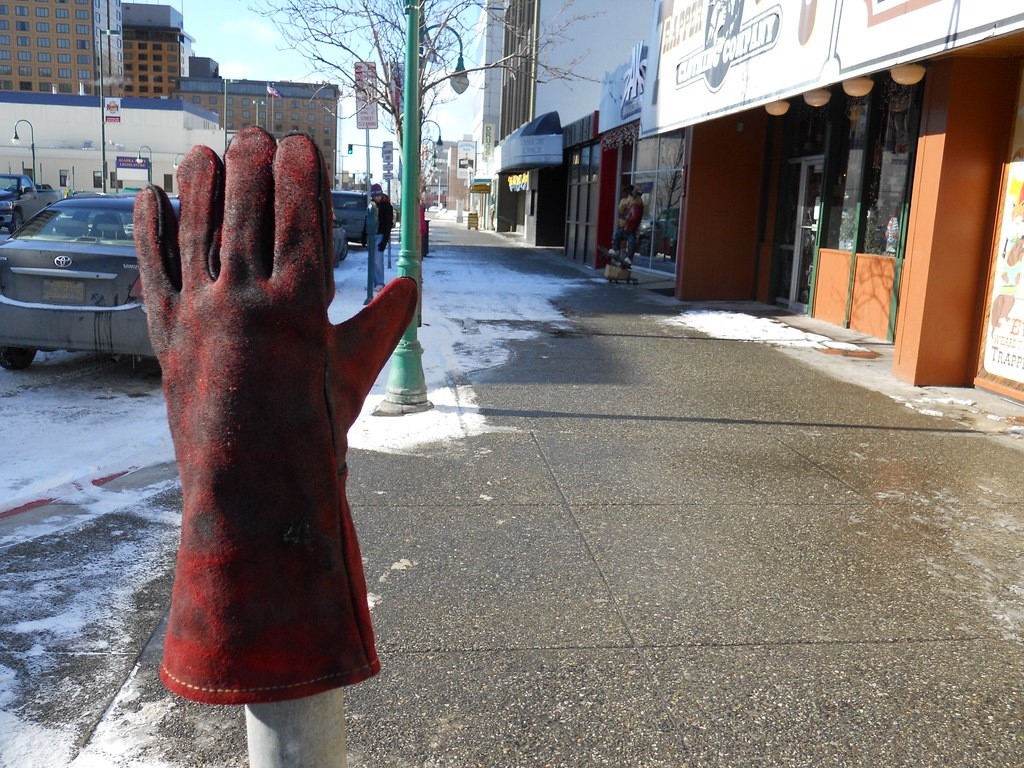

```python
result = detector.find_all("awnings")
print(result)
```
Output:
[493,110,563,175]
[468,175,492,194]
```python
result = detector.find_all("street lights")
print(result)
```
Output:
[135,144,153,186]
[12,119,36,185]
[97,28,121,195]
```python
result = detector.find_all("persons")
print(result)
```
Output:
[420,201,427,261]
[362,184,393,291]
[608,185,644,264]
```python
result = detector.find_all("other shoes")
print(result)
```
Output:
[373,284,384,291]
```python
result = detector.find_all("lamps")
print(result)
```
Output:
[841,74,874,97]
[801,86,831,107]
[764,98,790,116]
[889,59,926,85]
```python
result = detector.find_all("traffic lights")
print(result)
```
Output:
[347,144,352,154]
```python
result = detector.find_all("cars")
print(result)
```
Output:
[35,183,58,209]
[0,173,40,234]
[0,191,177,379]
[65,187,143,199]
[635,206,680,258]
[332,211,349,269]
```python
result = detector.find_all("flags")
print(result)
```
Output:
[267,83,281,98]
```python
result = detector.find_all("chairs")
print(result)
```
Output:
[87,213,127,240]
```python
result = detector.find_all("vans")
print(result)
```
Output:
[330,189,369,246]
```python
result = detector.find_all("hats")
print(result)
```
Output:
[371,184,382,196]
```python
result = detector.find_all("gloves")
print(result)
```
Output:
[377,241,386,251]
[361,236,367,246]
[132,126,419,703]
